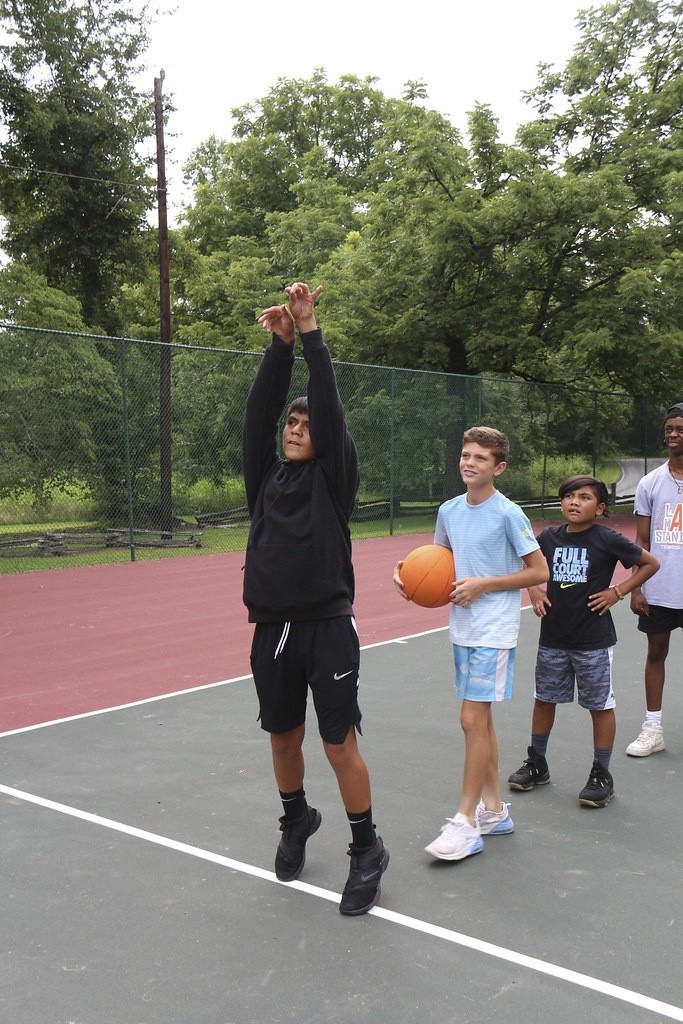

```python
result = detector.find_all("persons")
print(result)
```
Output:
[394,424,549,862]
[625,401,683,757]
[508,474,665,809]
[240,282,389,917]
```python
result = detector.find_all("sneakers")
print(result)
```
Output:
[625,720,666,757]
[339,829,390,916]
[439,797,515,835]
[508,745,551,791]
[275,805,322,882]
[577,758,616,807]
[423,813,485,861]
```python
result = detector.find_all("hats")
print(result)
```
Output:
[666,402,683,418]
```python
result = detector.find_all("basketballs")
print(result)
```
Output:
[399,544,456,608]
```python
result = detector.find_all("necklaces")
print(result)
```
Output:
[669,464,683,494]
[669,466,683,474]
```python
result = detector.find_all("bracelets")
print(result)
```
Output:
[614,585,624,600]
